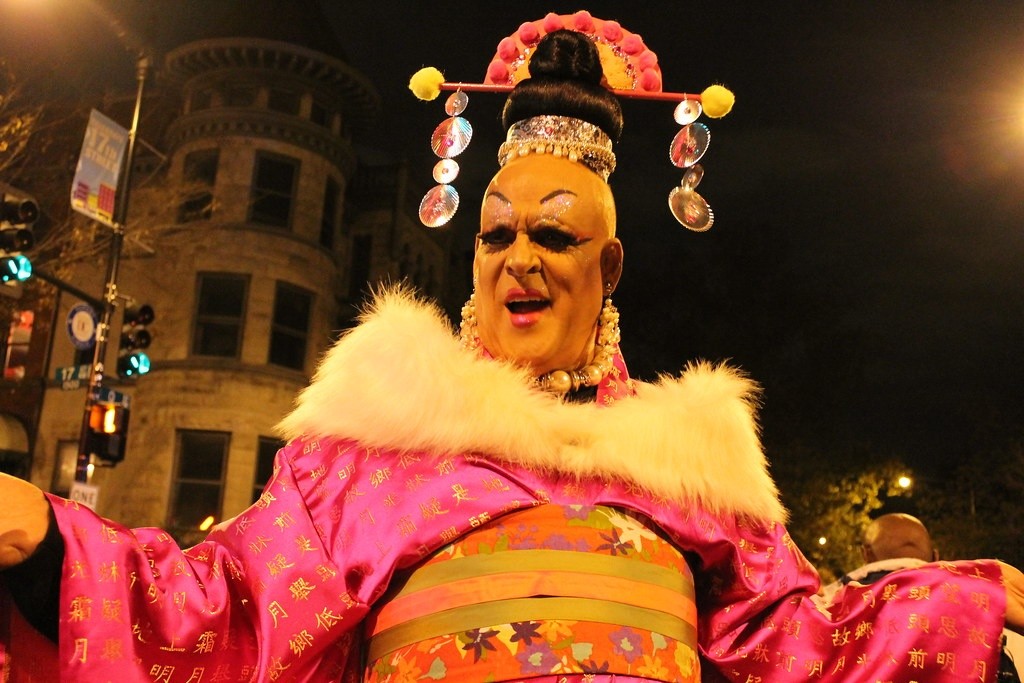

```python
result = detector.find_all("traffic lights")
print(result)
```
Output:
[114,301,157,380]
[90,404,131,460]
[0,192,43,285]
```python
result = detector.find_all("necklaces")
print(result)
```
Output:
[461,292,620,391]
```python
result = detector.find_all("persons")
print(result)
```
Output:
[839,513,939,586]
[0,28,1024,683]
